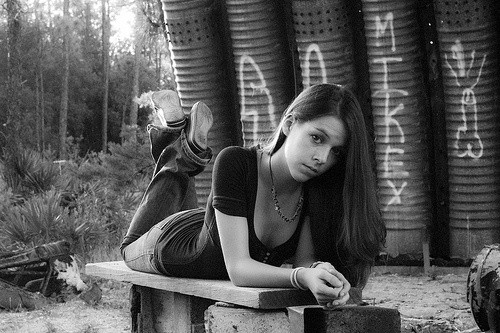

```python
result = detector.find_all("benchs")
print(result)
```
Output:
[84,260,364,332]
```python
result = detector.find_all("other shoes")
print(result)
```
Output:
[184,101,214,156]
[151,89,186,128]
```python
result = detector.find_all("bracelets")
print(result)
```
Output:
[290,267,304,290]
[309,261,323,268]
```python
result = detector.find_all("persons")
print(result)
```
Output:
[120,84,384,308]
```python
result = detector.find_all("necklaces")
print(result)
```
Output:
[267,149,304,223]
